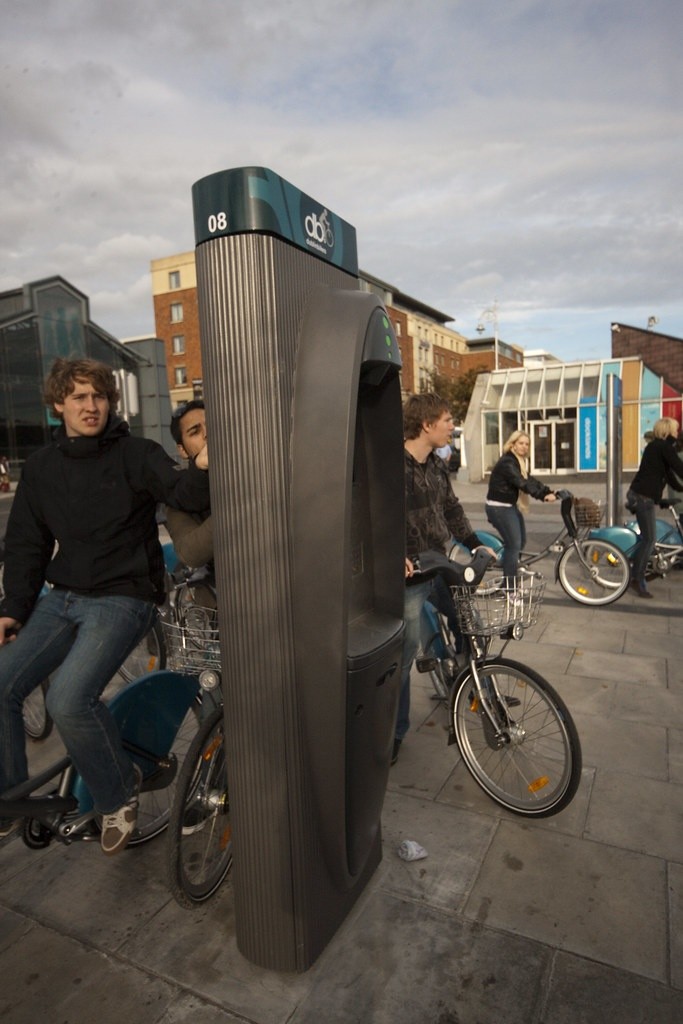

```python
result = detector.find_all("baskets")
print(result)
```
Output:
[156,599,223,675]
[451,570,545,636]
[576,495,609,527]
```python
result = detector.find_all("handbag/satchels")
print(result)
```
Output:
[159,574,220,670]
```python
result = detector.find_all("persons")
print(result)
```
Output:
[0,360,210,855]
[486,430,557,594]
[166,400,216,836]
[625,416,683,598]
[390,393,519,766]
[436,445,453,468]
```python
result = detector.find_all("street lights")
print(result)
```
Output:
[477,308,499,368]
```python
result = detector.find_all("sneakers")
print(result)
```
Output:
[0,816,26,842]
[99,764,143,856]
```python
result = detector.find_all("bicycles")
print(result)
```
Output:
[404,547,582,819]
[157,567,237,913]
[0,670,214,859]
[580,497,683,583]
[447,490,630,612]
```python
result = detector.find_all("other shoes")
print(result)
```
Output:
[631,577,655,599]
[502,586,523,607]
[390,739,402,764]
[468,689,521,707]
